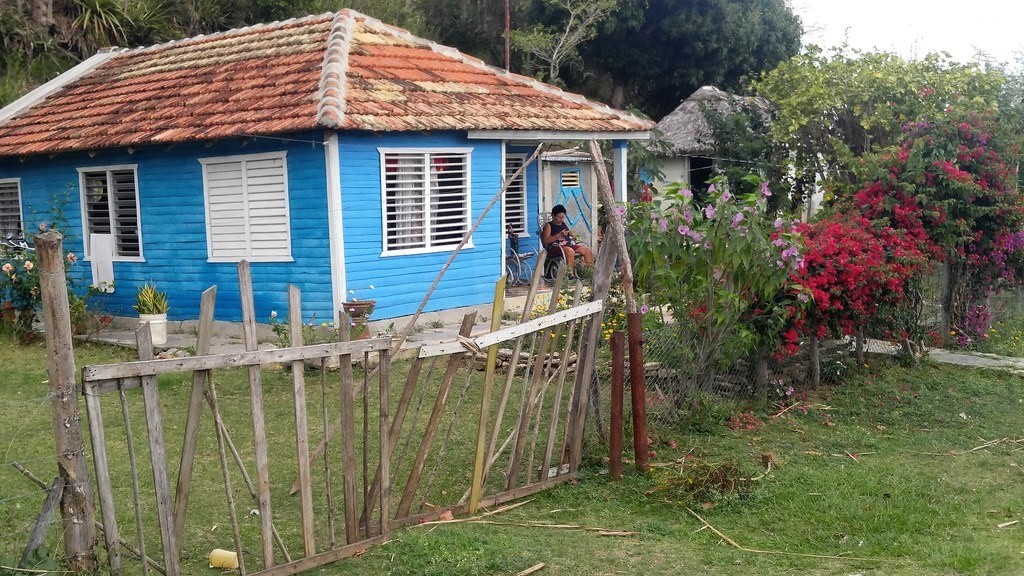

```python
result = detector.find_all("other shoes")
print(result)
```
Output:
[569,276,578,280]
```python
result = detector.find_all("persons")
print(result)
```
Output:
[542,204,592,280]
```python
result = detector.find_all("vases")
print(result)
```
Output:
[510,234,518,254]
[341,302,376,317]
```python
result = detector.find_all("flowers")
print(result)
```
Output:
[348,284,374,301]
[508,223,520,246]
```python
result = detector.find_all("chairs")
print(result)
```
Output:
[506,244,539,286]
[537,212,592,284]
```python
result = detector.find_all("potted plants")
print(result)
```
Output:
[131,281,172,345]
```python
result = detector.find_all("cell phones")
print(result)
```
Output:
[565,229,569,231]
